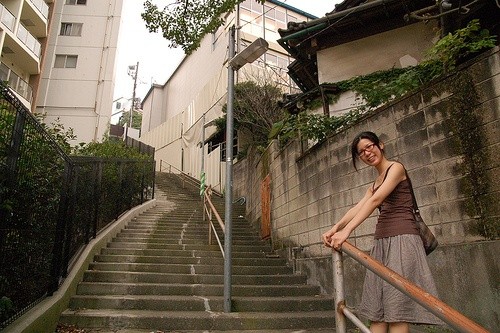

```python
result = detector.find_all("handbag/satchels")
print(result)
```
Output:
[414,212,438,256]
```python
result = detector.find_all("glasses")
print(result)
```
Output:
[358,143,376,156]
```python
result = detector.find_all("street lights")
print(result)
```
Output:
[222,25,270,312]
[125,60,138,131]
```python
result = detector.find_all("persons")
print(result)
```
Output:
[322,131,445,333]
